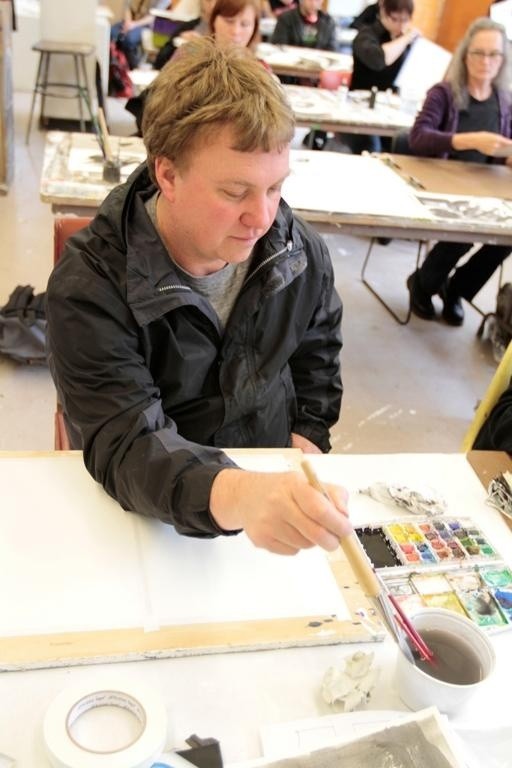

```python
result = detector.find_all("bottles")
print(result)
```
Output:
[334,78,349,114]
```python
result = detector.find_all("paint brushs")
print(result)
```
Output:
[77,85,112,162]
[300,458,440,669]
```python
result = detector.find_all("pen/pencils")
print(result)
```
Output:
[410,176,426,191]
[387,159,401,169]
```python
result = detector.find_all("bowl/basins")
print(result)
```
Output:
[391,608,499,722]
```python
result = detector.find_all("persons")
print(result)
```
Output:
[349,0,416,154]
[407,17,512,325]
[46,34,351,555]
[112,0,340,84]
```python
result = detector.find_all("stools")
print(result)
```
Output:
[25,39,93,143]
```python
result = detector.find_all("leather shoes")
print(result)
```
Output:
[407,269,436,319]
[439,278,464,325]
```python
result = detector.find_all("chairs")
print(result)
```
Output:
[52,217,98,450]
[361,129,504,325]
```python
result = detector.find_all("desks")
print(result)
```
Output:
[40,129,511,248]
[135,68,422,137]
[0,449,512,764]
[256,16,359,80]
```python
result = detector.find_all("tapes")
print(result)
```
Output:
[43,677,167,768]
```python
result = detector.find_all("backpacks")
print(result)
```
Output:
[1,284,47,368]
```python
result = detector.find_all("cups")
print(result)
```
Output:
[103,137,121,184]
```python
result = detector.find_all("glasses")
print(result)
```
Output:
[467,50,503,61]
